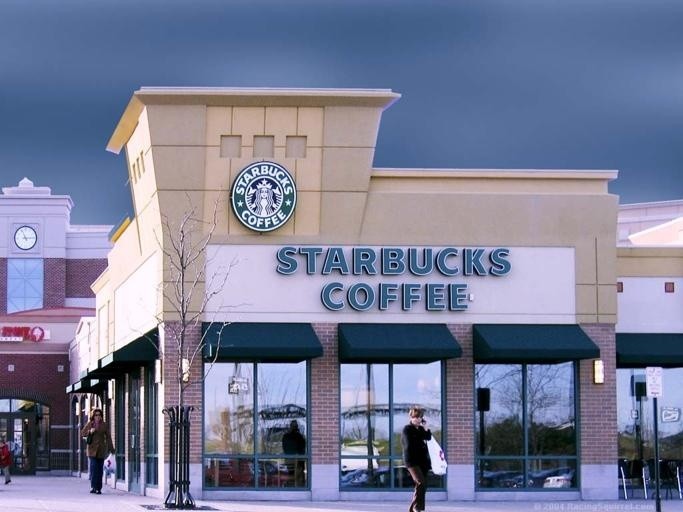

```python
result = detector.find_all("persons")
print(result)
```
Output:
[0,441,13,484]
[400,408,431,512]
[282,420,306,475]
[81,409,115,494]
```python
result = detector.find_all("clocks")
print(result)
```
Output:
[14,226,37,250]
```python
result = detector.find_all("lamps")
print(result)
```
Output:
[182,358,190,383]
[592,360,605,384]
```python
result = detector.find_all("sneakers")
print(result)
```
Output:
[90,488,96,493]
[5,481,8,484]
[8,480,11,482]
[96,490,101,494]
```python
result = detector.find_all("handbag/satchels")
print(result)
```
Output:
[87,432,95,444]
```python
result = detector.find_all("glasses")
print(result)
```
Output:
[95,414,102,416]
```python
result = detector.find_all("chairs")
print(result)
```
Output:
[618,457,683,500]
[272,459,298,488]
